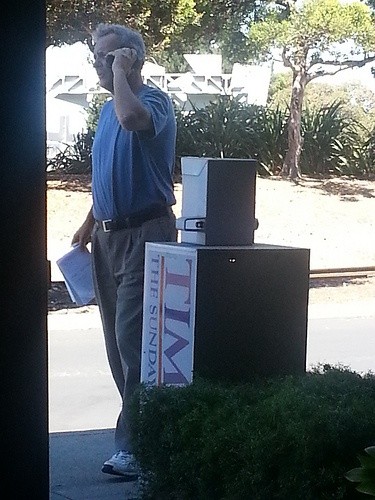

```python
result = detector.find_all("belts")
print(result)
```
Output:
[96,206,172,233]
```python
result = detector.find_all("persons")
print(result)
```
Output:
[72,25,177,476]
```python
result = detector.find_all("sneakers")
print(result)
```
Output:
[101,451,136,476]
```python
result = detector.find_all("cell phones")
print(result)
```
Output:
[106,54,143,71]
